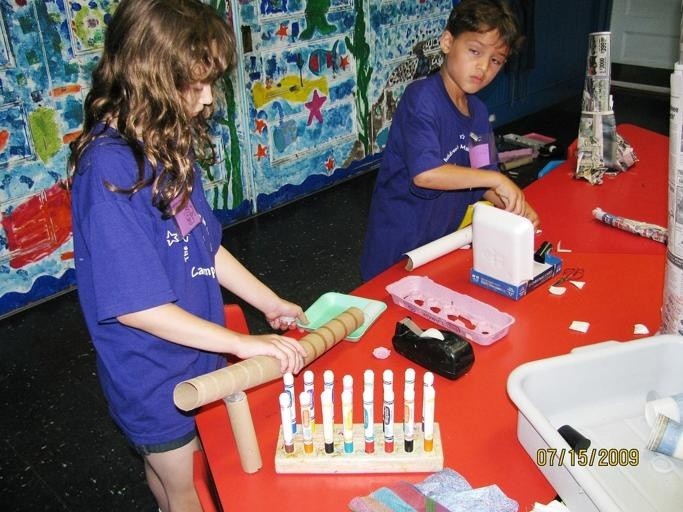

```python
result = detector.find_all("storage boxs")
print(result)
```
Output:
[505,332,683,512]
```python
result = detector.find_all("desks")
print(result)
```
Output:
[193,124,683,512]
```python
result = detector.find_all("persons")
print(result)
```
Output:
[66,0,311,511]
[356,0,541,285]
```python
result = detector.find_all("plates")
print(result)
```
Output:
[296,291,388,342]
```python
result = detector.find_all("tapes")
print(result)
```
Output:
[399,316,445,342]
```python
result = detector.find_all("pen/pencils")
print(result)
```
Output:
[279,368,442,459]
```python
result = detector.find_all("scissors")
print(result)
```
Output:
[552,268,585,288]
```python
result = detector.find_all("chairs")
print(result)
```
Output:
[193,304,251,512]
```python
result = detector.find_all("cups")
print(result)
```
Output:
[645,392,682,459]
